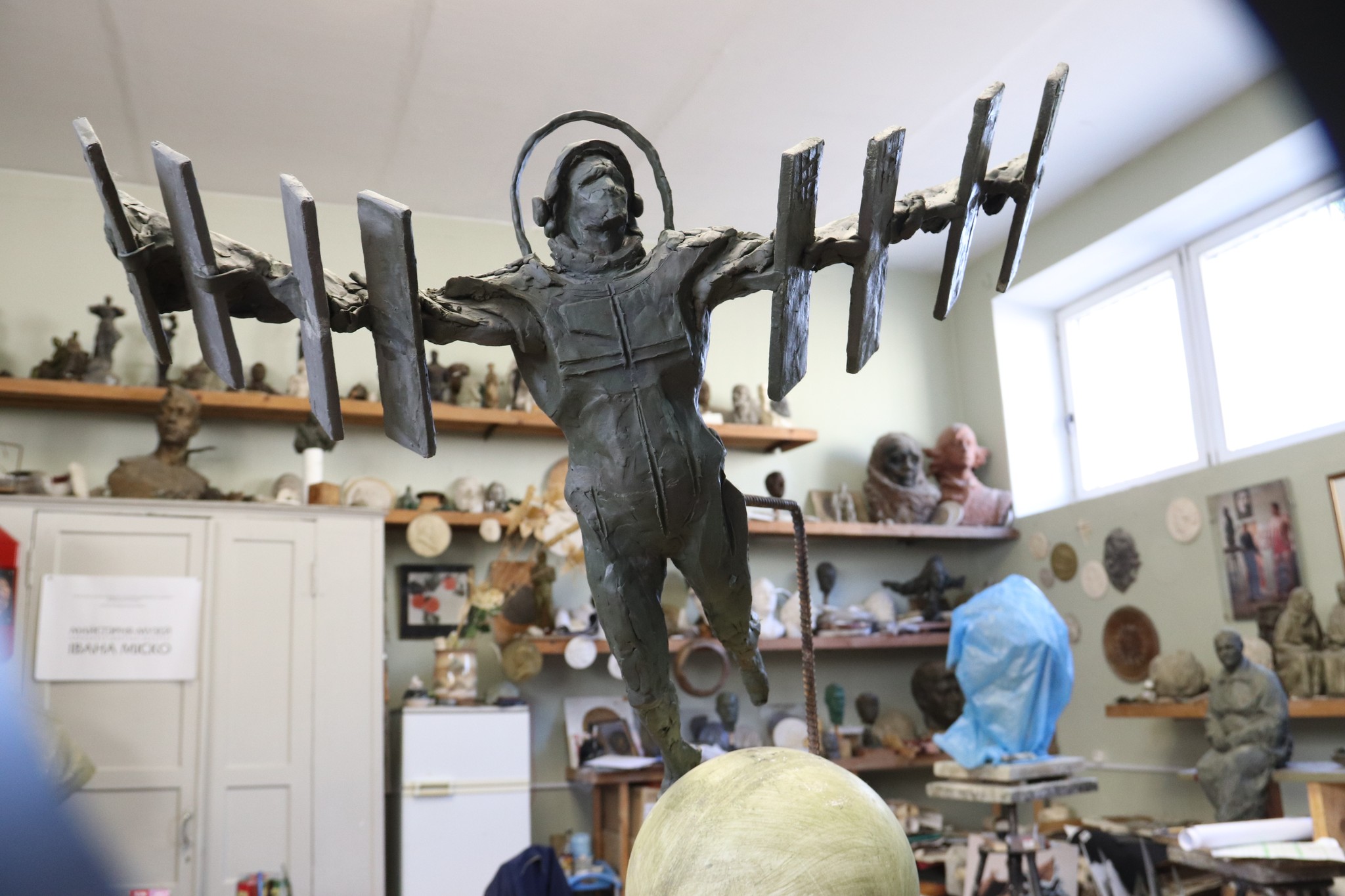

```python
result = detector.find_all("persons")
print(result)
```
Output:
[526,552,557,635]
[103,139,1041,798]
[1238,499,1294,602]
[1194,631,1293,821]
[856,692,880,725]
[866,433,942,525]
[832,481,860,523]
[924,421,1012,528]
[884,552,965,615]
[1275,588,1326,697]
[1324,584,1345,696]
[426,349,448,400]
[481,363,499,408]
[87,297,125,371]
[698,692,739,747]
[117,379,211,499]
[910,660,964,729]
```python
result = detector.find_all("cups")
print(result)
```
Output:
[433,649,478,705]
[571,832,593,873]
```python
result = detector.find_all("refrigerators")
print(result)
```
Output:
[400,703,533,896]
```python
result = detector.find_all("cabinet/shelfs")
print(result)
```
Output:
[1104,699,1345,896]
[562,743,1069,896]
[0,498,389,896]
[0,375,1020,655]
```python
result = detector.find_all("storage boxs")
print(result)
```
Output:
[598,786,663,866]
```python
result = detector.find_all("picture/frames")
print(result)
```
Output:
[398,561,474,639]
